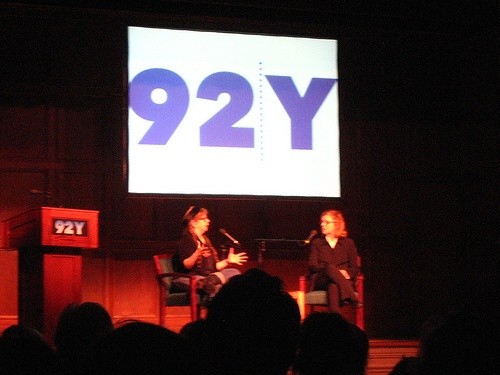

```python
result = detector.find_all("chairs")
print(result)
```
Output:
[152,253,216,328]
[298,255,363,333]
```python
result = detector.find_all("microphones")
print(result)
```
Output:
[305,230,317,244]
[30,189,53,196]
[219,229,239,245]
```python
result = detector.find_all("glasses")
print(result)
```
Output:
[321,220,337,224]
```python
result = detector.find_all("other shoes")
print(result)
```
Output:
[346,298,363,308]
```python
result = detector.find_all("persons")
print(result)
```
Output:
[1,271,499,374]
[304,209,365,313]
[172,204,249,303]
